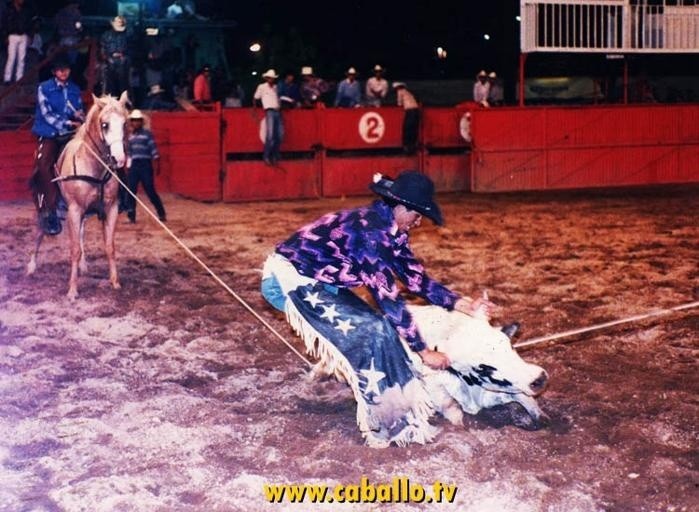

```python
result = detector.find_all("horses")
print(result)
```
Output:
[24,90,129,304]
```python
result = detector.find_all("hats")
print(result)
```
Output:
[49,51,77,67]
[301,66,313,76]
[344,67,358,76]
[372,64,384,72]
[261,69,280,79]
[369,170,444,226]
[391,80,407,90]
[109,14,128,32]
[127,109,149,119]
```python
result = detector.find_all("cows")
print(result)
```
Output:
[306,281,552,445]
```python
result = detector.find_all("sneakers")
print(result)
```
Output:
[47,212,61,235]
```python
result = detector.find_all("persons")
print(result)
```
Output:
[297,66,328,101]
[278,73,300,108]
[393,81,422,156]
[101,16,135,97]
[252,68,284,165]
[32,54,84,235]
[473,71,500,107]
[1,0,100,80]
[259,169,495,443]
[179,63,246,103]
[366,64,390,104]
[147,84,178,111]
[487,71,503,104]
[333,68,362,106]
[125,110,167,223]
